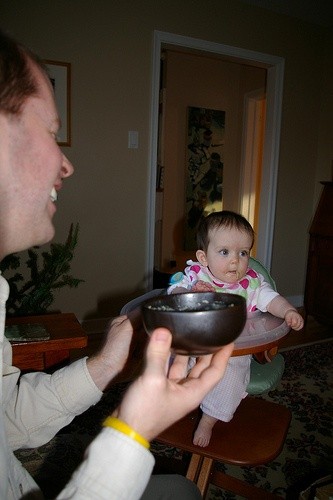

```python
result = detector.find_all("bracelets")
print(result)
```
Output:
[102,418,151,448]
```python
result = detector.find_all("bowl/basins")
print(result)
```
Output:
[140,292,247,356]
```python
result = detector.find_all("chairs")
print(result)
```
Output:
[99,259,294,500]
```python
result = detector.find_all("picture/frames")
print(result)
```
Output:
[41,59,71,147]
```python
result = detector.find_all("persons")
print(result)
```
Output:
[166,210,303,447]
[0,31,234,500]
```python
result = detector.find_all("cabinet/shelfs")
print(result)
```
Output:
[4,313,88,375]
